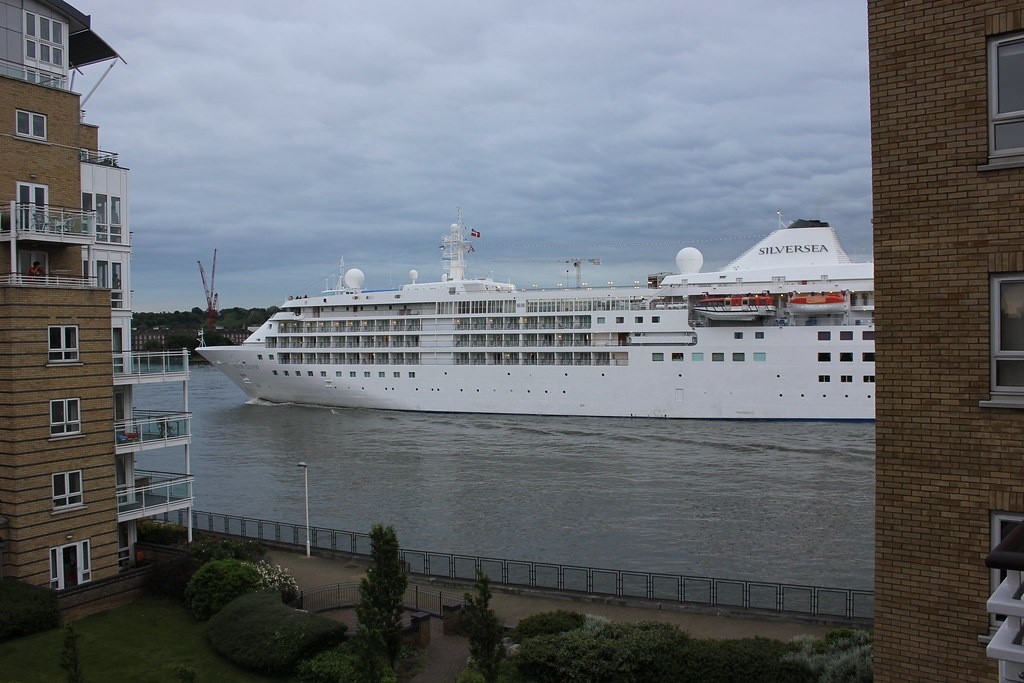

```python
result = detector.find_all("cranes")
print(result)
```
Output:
[196,248,221,331]
[491,256,602,288]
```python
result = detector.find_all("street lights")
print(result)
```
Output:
[297,461,311,557]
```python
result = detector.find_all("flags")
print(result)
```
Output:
[471,228,480,237]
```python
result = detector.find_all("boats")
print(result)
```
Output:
[691,289,777,322]
[787,294,849,316]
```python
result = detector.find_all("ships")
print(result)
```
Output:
[194,204,875,423]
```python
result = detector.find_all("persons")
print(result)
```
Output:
[28,261,46,283]
[113,274,121,308]
[97,204,119,243]
